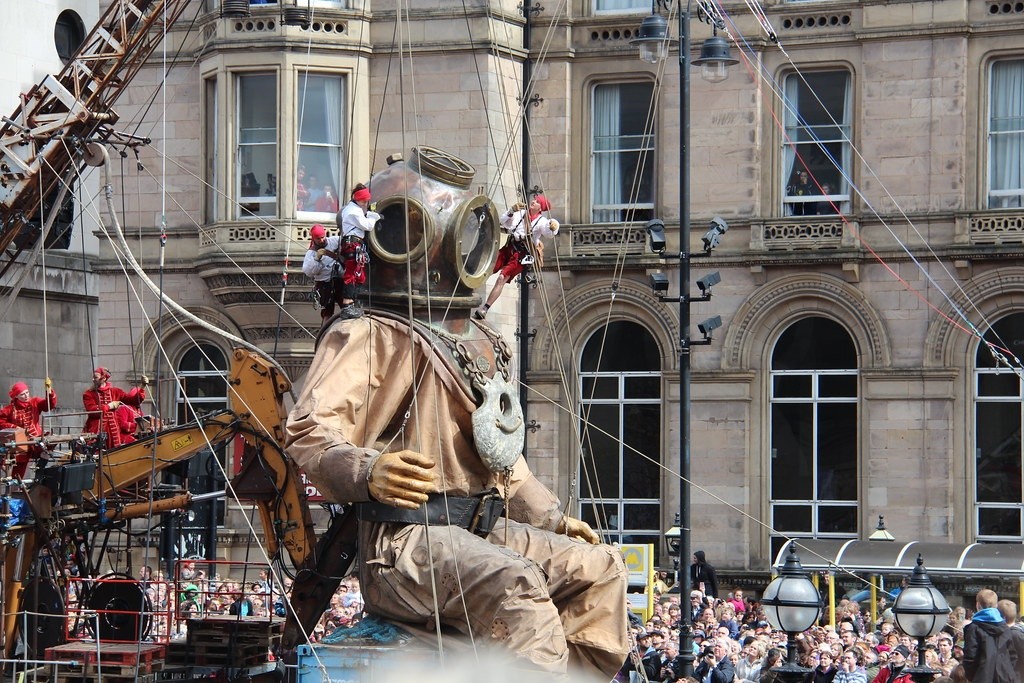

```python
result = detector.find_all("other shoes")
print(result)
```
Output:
[475,306,488,319]
[340,304,363,319]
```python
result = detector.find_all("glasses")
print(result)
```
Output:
[891,651,900,657]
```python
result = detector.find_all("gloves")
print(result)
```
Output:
[549,219,557,232]
[314,249,326,262]
[110,401,121,408]
[44,376,51,393]
[512,202,526,212]
[140,375,149,389]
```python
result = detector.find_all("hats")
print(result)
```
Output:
[258,588,266,594]
[893,645,911,658]
[758,620,768,628]
[695,629,705,639]
[71,567,78,574]
[777,643,787,649]
[651,630,665,639]
[635,633,651,640]
[313,624,325,636]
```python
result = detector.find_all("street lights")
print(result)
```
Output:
[634,0,738,683]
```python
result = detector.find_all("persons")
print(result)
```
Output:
[787,169,841,216]
[610,551,789,683]
[342,183,380,320]
[140,562,211,643]
[963,589,1024,683]
[297,164,339,213]
[52,569,95,632]
[308,571,369,643]
[209,569,295,620]
[795,571,970,683]
[0,378,58,480]
[474,194,559,318]
[80,367,149,455]
[302,224,346,323]
[284,144,632,682]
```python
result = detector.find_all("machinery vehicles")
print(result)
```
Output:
[1,0,461,683]
